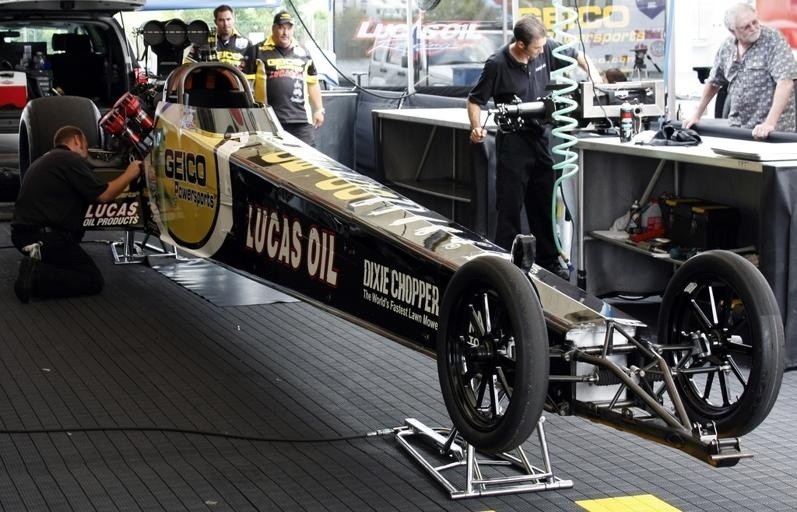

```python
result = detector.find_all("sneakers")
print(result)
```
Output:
[14,258,38,306]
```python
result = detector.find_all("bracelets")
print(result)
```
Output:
[311,108,326,116]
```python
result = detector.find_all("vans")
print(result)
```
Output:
[0,0,149,166]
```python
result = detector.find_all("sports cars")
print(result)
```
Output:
[18,59,787,456]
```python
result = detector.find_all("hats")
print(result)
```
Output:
[274,12,295,26]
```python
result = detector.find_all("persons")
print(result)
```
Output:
[463,11,608,281]
[236,7,326,150]
[178,4,255,72]
[679,0,797,143]
[8,124,146,304]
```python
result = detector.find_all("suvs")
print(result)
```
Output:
[367,26,517,88]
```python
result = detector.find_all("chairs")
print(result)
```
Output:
[51,31,104,92]
